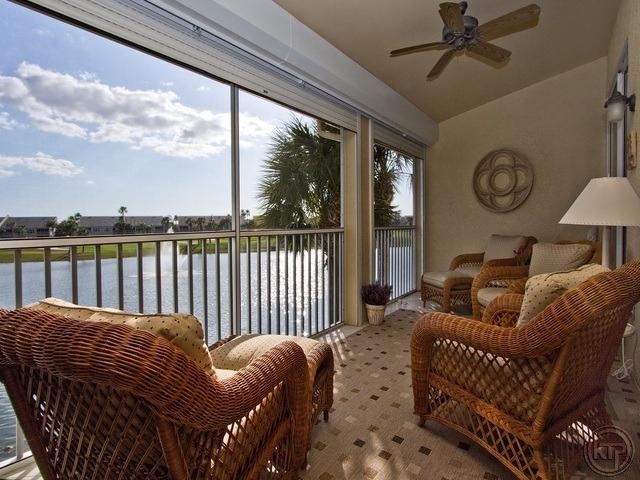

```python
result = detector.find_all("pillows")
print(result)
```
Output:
[482,234,528,265]
[27,296,217,378]
[516,263,609,326]
[528,242,594,277]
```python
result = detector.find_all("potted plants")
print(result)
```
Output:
[361,282,394,326]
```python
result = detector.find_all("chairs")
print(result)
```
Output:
[1,296,309,480]
[451,235,538,307]
[472,240,603,321]
[410,261,640,479]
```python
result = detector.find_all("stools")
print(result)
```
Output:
[207,334,334,449]
[421,268,474,313]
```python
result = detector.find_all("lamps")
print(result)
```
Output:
[604,83,635,123]
[558,175,640,265]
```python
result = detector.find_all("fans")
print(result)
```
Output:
[390,1,541,80]
